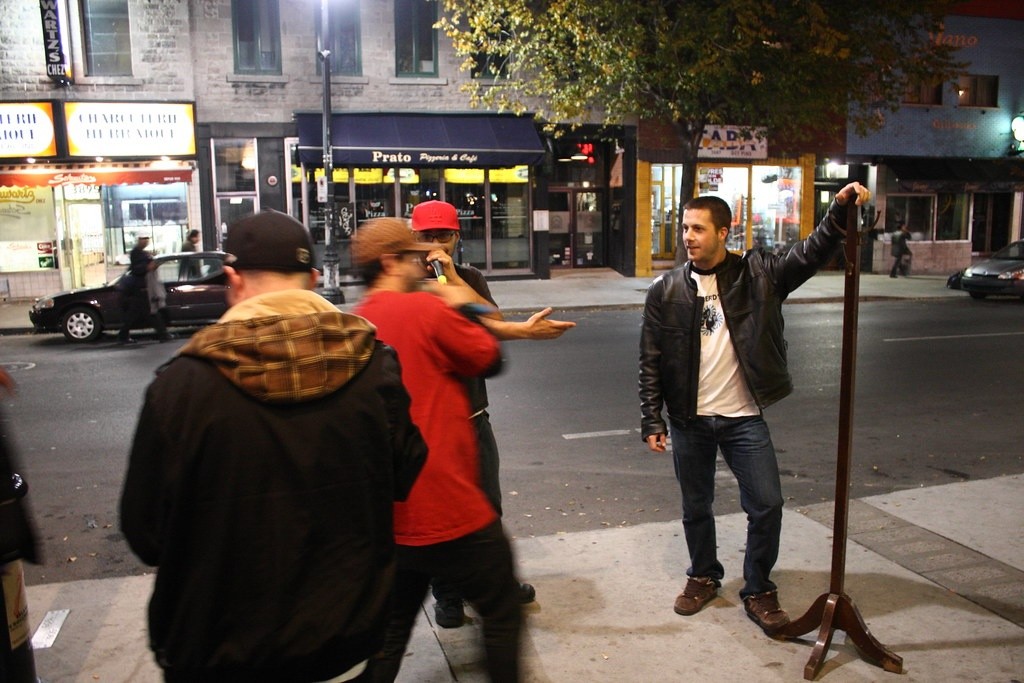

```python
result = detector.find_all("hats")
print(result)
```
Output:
[411,201,463,233]
[348,214,443,256]
[220,206,319,273]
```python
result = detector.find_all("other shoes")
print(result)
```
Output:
[743,591,792,633]
[672,575,721,616]
[518,583,536,603]
[117,337,138,344]
[890,274,898,278]
[160,333,180,343]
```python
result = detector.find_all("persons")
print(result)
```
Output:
[118,205,429,683]
[889,220,912,278]
[412,201,576,628]
[178,230,202,282]
[119,233,180,344]
[346,216,522,683]
[640,181,871,630]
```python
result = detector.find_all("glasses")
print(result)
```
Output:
[417,230,456,243]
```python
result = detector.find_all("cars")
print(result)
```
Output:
[27,251,345,345]
[946,239,1024,301]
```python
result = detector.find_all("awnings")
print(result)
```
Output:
[884,159,1024,192]
[0,160,193,188]
[295,113,546,168]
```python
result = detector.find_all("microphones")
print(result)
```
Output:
[430,259,447,285]
